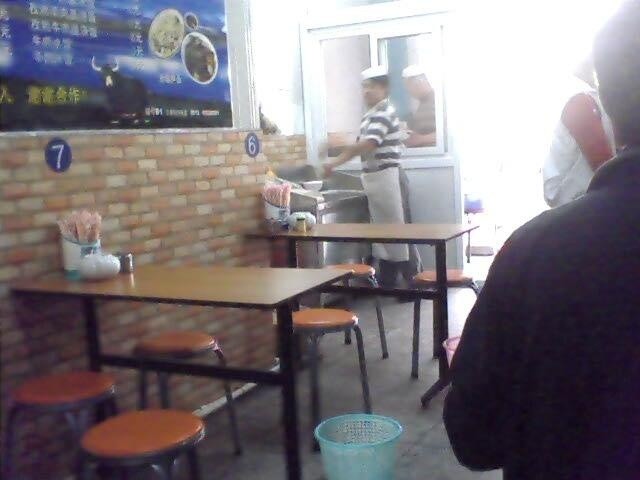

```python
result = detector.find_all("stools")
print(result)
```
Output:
[412,271,480,377]
[0,370,126,480]
[292,308,372,452]
[72,409,207,480]
[132,332,243,457]
[323,264,389,359]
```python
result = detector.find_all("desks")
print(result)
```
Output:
[11,264,354,480]
[245,223,479,407]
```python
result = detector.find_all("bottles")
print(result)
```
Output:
[327,129,353,146]
[294,216,307,233]
[269,217,278,234]
[124,251,135,272]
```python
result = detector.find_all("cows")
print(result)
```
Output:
[91,56,155,129]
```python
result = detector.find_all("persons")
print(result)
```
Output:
[543,88,617,209]
[318,65,425,303]
[401,65,435,147]
[444,0,640,480]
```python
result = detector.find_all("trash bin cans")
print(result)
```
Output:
[314,413,403,480]
[442,336,461,368]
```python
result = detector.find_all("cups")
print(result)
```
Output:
[58,235,101,279]
[262,199,292,227]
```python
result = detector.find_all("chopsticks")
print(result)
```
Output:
[266,188,290,208]
[60,217,101,244]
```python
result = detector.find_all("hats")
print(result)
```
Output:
[401,64,425,79]
[361,65,389,81]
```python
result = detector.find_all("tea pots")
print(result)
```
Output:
[74,252,121,280]
[285,210,317,230]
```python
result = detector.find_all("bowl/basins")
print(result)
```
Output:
[181,31,219,85]
[302,181,323,191]
[184,11,199,32]
[147,8,185,59]
